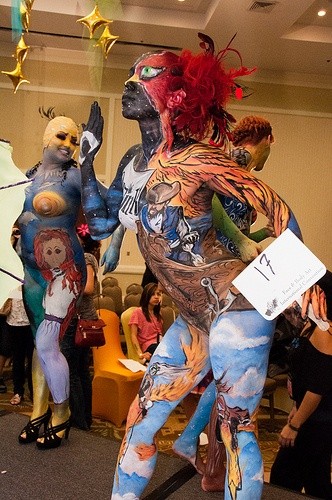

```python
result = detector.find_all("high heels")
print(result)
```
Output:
[18,404,71,449]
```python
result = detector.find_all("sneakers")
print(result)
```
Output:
[0,376,8,393]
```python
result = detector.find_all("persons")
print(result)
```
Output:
[61,241,103,432]
[80,50,329,500]
[7,284,33,406]
[173,117,275,491]
[11,115,125,450]
[129,284,164,360]
[270,270,332,500]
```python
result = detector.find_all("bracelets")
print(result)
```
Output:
[288,423,299,432]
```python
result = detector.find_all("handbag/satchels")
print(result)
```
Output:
[74,318,107,347]
[0,298,13,316]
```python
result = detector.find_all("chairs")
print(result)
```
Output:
[92,276,179,428]
[262,376,289,427]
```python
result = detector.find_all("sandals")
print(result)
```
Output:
[10,393,21,405]
